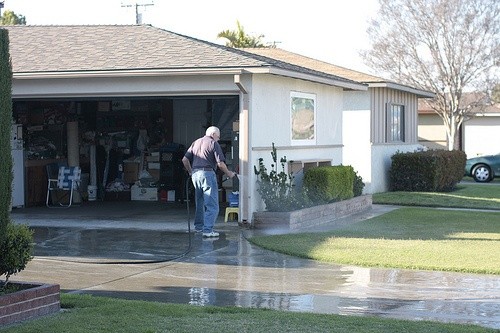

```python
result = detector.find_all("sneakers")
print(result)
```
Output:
[195,227,203,232]
[202,231,219,236]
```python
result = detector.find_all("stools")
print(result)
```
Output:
[224,207,239,223]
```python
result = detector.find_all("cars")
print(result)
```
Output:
[464,153,500,183]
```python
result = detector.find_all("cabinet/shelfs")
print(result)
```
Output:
[24,157,67,206]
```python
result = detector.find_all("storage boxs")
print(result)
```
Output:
[131,187,159,201]
[96,98,148,112]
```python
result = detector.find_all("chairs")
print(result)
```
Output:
[47,165,82,208]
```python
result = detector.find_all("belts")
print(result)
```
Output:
[198,167,213,171]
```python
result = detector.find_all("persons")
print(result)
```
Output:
[182,126,235,237]
